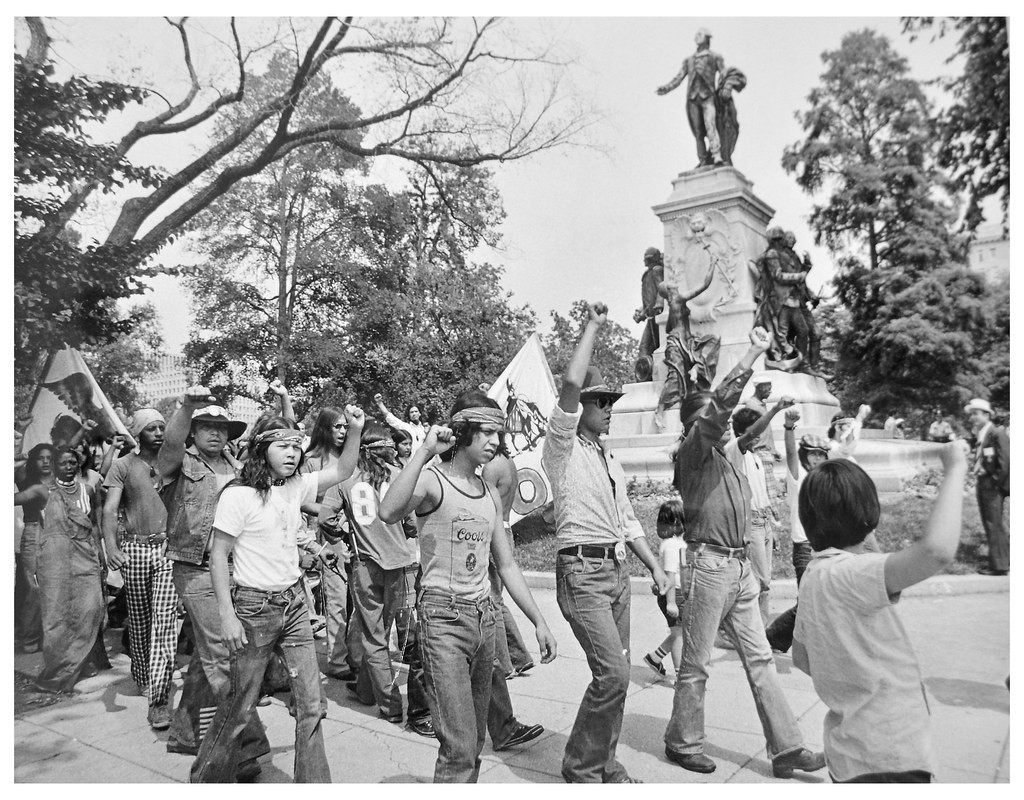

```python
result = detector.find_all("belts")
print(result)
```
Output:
[559,545,615,558]
[688,543,743,558]
[125,533,167,546]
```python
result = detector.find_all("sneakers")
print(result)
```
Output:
[772,748,826,777]
[665,745,716,772]
[644,653,666,677]
[503,724,544,746]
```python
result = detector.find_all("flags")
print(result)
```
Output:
[474,334,559,527]
[21,341,125,454]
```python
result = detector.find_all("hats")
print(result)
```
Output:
[752,377,772,386]
[191,394,247,441]
[964,399,995,416]
[800,435,831,453]
[581,366,626,404]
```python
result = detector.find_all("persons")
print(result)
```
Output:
[191,402,365,784]
[929,411,953,442]
[665,325,826,778]
[14,380,543,749]
[654,253,719,428]
[545,301,673,784]
[724,395,794,649]
[790,438,971,784]
[633,246,664,356]
[379,395,559,783]
[885,408,898,438]
[964,398,1010,575]
[761,225,836,382]
[657,26,724,166]
[645,500,688,681]
[747,376,872,652]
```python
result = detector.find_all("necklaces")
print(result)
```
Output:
[266,497,287,530]
[272,478,285,486]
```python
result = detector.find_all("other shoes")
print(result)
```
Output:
[256,696,272,706]
[347,682,356,691]
[148,705,172,728]
[380,709,402,722]
[83,662,98,676]
[136,683,150,696]
[327,670,356,681]
[978,567,1007,575]
[167,736,199,754]
[24,642,39,652]
[407,719,435,734]
[237,759,262,780]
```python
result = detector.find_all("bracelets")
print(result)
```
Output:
[783,424,795,431]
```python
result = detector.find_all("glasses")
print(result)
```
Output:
[332,423,349,429]
[581,396,613,408]
